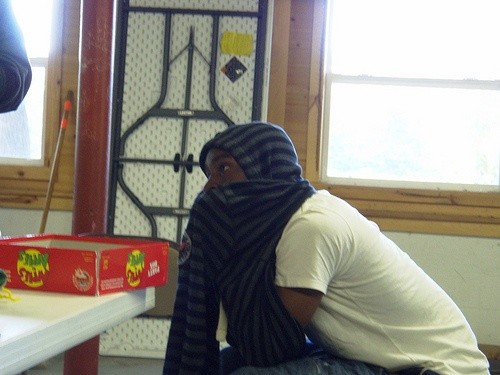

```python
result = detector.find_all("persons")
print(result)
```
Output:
[0,1,33,114]
[178,124,493,375]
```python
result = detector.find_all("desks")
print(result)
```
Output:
[0,287,156,375]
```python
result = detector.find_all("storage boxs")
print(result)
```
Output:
[1,232,170,297]
